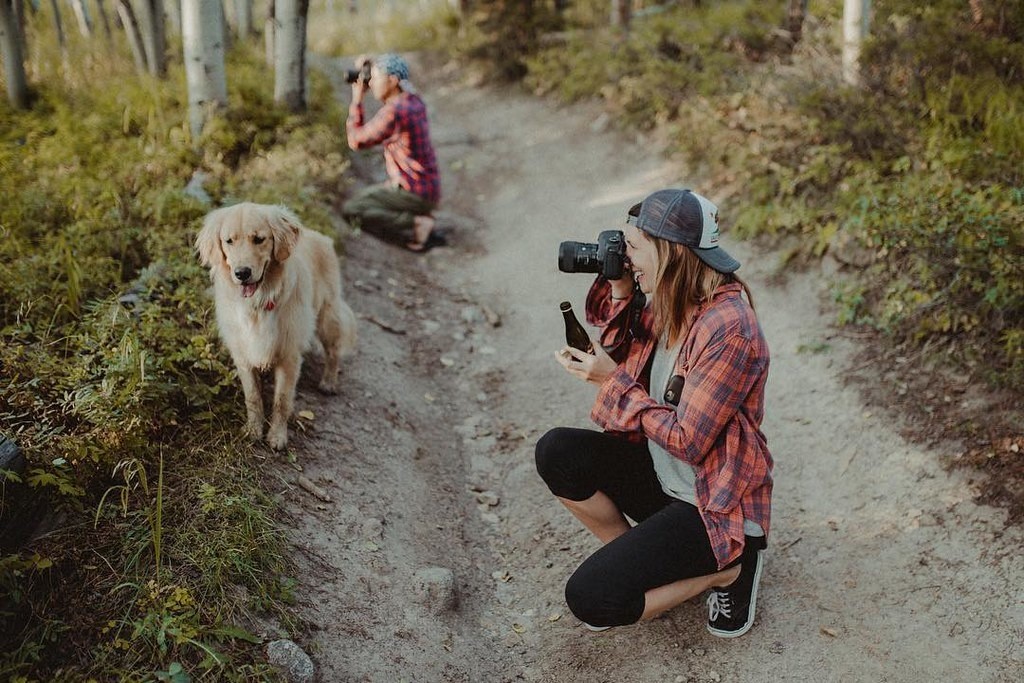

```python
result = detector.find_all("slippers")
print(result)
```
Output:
[405,231,447,253]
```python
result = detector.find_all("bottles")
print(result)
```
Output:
[559,301,596,362]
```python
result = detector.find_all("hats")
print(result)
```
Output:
[371,53,418,95]
[626,188,741,275]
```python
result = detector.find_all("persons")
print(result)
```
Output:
[534,189,774,639]
[341,55,441,251]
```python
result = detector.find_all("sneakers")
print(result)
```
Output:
[704,544,763,638]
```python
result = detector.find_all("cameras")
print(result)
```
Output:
[557,230,628,280]
[344,64,372,87]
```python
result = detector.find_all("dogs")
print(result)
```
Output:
[195,202,357,453]
